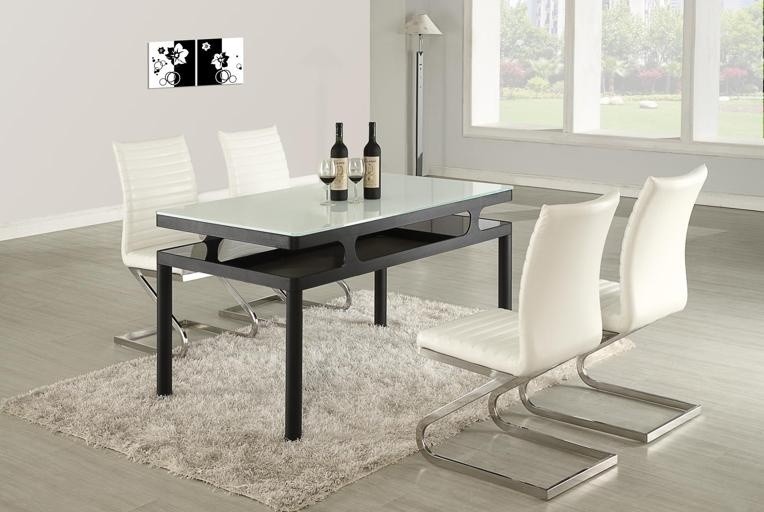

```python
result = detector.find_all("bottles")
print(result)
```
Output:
[330,122,348,201]
[363,121,381,200]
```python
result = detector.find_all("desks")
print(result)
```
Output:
[156,172,513,441]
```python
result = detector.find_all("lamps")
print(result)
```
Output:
[396,14,443,176]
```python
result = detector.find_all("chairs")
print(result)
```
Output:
[113,136,259,359]
[518,163,709,445]
[217,126,356,326]
[416,187,620,500]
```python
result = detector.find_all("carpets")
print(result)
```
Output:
[0,288,636,512]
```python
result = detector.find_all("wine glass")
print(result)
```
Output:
[346,159,365,203]
[319,159,338,206]
